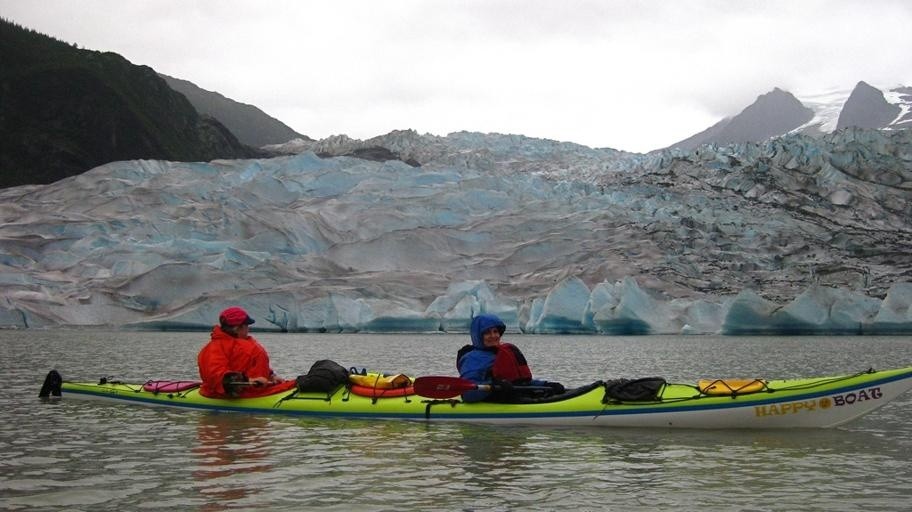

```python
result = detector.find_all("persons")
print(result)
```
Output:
[197,307,287,398]
[456,314,566,402]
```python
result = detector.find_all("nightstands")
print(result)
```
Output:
[413,376,570,398]
[222,372,279,397]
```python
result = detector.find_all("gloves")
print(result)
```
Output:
[40,367,912,428]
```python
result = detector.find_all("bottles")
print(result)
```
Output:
[220,307,255,326]
